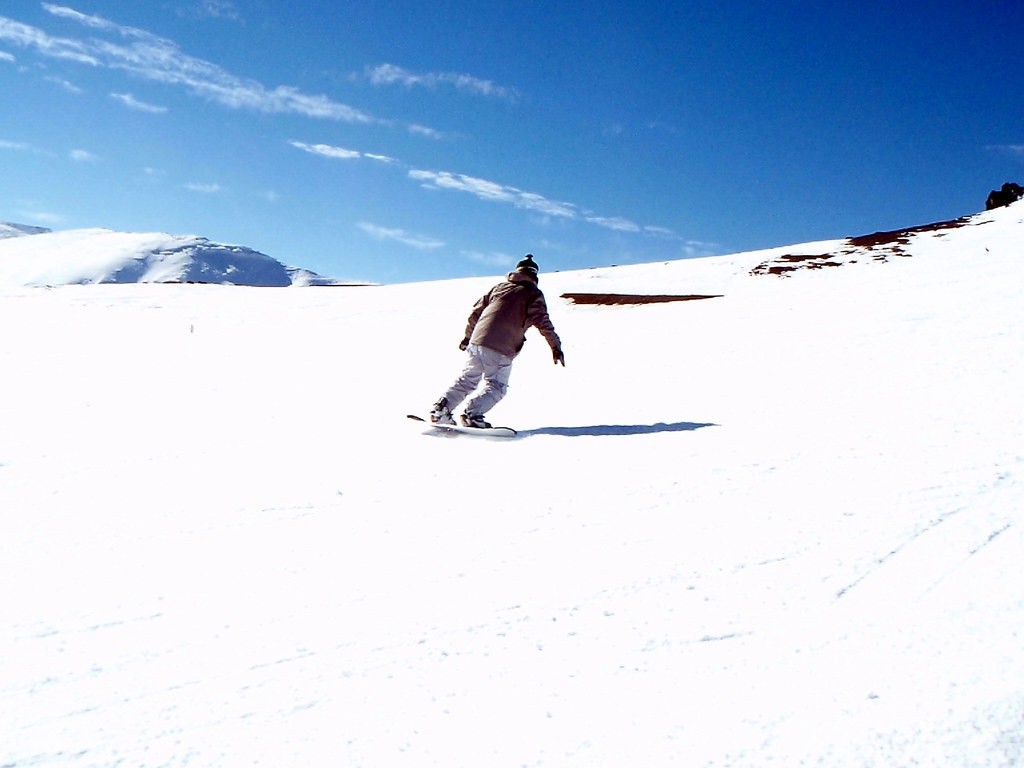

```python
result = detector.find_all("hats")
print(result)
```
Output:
[516,254,539,276]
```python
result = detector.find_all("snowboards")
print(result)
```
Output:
[407,415,517,437]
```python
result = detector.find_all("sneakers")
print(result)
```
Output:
[431,398,457,425]
[460,411,492,428]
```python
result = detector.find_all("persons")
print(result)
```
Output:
[431,254,565,428]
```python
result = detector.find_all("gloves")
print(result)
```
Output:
[552,346,565,367]
[459,337,470,351]
